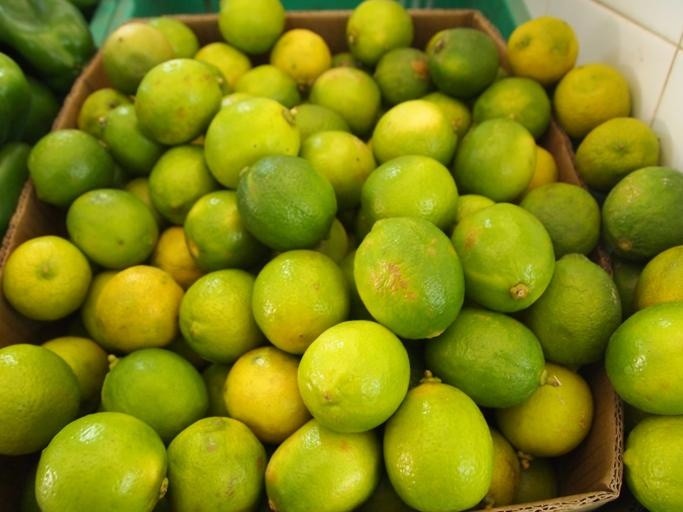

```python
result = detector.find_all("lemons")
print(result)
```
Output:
[0,0,682,512]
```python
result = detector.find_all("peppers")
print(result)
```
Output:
[1,1,99,246]
